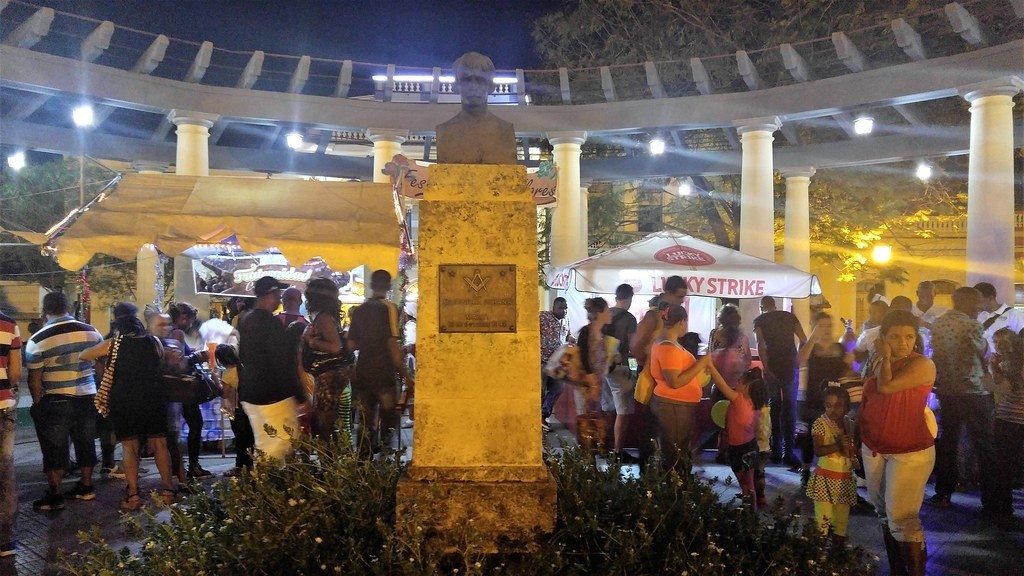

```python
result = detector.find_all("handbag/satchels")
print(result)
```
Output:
[94,335,122,418]
[544,345,589,387]
[190,353,221,405]
[633,367,654,405]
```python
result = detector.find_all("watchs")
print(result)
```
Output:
[878,354,890,362]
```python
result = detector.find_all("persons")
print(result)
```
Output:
[78,298,254,510]
[437,52,517,165]
[806,379,862,554]
[853,309,936,575]
[348,269,412,461]
[850,280,1024,522]
[540,275,873,514]
[25,292,104,509]
[276,287,359,426]
[0,311,23,576]
[238,276,312,469]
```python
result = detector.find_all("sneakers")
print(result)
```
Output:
[64,482,96,500]
[33,494,65,510]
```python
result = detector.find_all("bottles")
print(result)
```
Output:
[838,320,860,373]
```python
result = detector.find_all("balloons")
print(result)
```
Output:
[711,400,731,428]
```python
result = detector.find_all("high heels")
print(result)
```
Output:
[120,496,140,512]
[161,488,181,506]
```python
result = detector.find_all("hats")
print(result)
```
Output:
[254,276,290,295]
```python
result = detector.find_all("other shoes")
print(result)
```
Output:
[224,464,248,475]
[0,542,15,556]
[736,491,743,498]
[923,494,949,507]
[757,496,764,505]
[801,469,810,490]
[189,465,213,479]
[611,451,639,464]
[541,417,551,433]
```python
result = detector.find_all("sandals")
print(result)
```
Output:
[101,465,128,480]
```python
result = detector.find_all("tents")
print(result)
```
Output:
[544,226,824,345]
[40,171,415,280]
